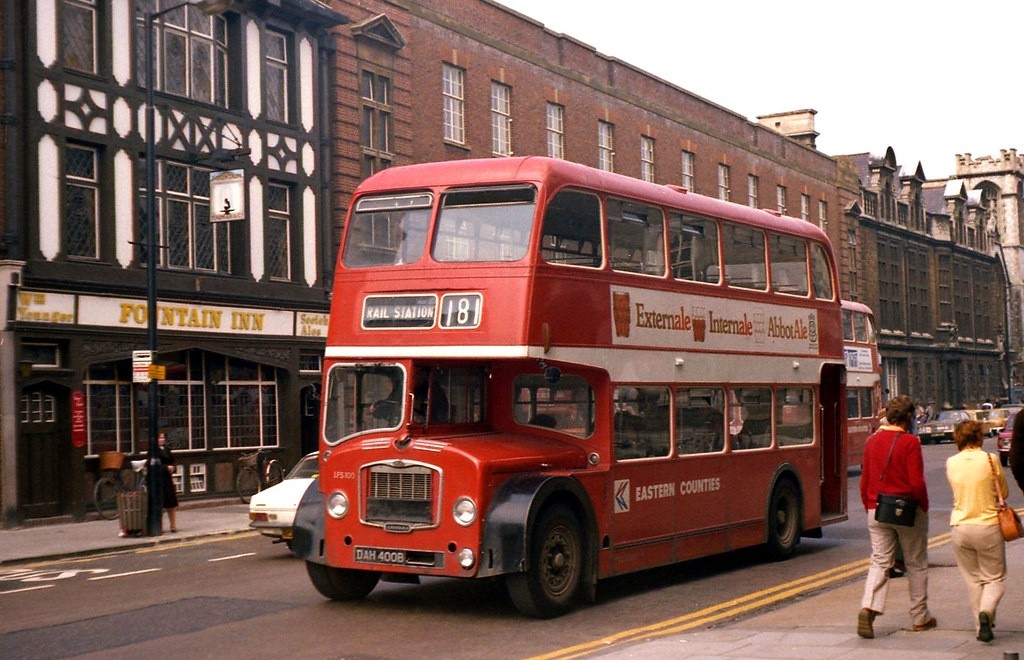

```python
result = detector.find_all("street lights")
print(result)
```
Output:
[144,0,236,535]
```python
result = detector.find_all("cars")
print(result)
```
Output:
[248,450,320,552]
[964,404,1024,438]
[919,410,971,446]
[996,412,1016,468]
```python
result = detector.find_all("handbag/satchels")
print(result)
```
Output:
[999,508,1024,541]
[875,495,919,528]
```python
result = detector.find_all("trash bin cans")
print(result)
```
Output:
[116,491,145,530]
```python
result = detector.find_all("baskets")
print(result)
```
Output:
[100,451,124,470]
[238,454,257,467]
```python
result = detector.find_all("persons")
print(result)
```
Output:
[857,394,937,639]
[737,407,771,449]
[993,402,1000,409]
[613,406,741,461]
[527,414,557,428]
[146,429,179,532]
[946,419,1024,642]
[369,366,449,427]
[982,398,993,409]
[1009,396,1024,495]
[942,400,956,410]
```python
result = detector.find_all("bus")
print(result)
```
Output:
[289,156,851,619]
[780,298,882,473]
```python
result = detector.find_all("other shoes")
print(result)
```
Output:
[888,569,904,578]
[913,617,937,632]
[856,607,875,639]
[977,611,994,643]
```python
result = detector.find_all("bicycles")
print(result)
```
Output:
[92,446,148,520]
[237,445,284,505]
